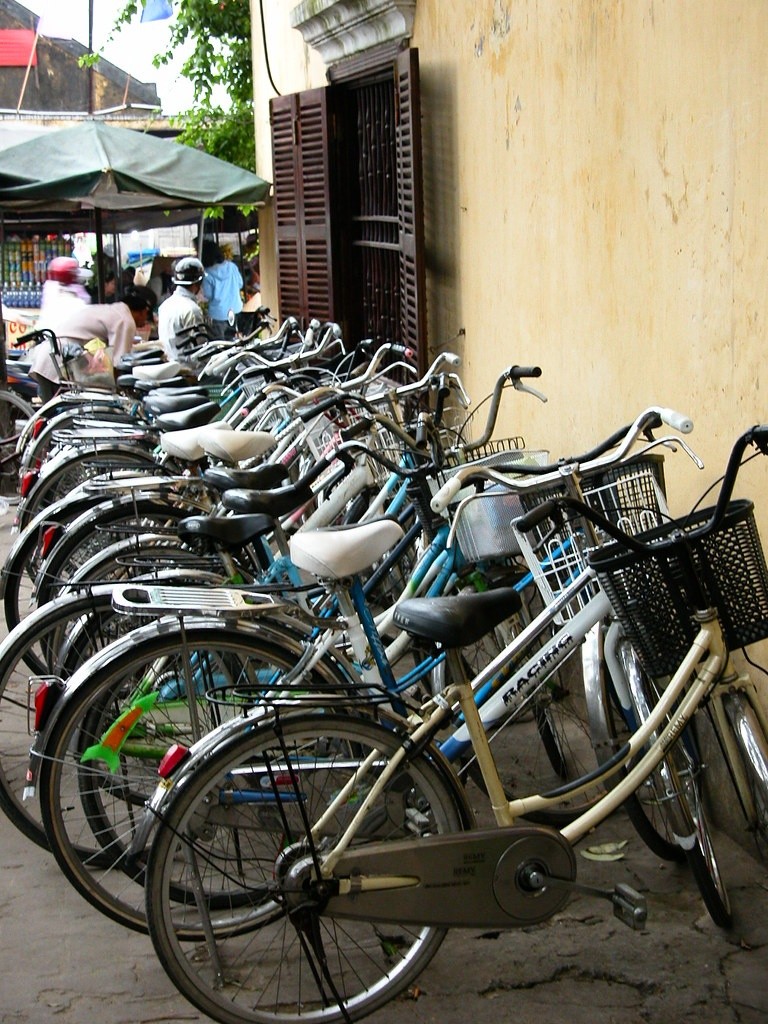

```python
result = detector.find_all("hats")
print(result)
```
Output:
[129,286,158,324]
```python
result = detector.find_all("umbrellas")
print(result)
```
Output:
[0,120,271,304]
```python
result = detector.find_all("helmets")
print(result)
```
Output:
[171,257,209,284]
[47,256,94,284]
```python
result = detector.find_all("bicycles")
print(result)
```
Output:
[0,306,767,1024]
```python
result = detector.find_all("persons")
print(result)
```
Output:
[28,233,261,404]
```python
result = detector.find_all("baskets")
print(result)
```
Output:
[587,499,768,679]
[221,334,574,564]
[517,454,668,596]
[66,345,117,393]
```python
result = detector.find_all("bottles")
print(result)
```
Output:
[0,234,74,308]
